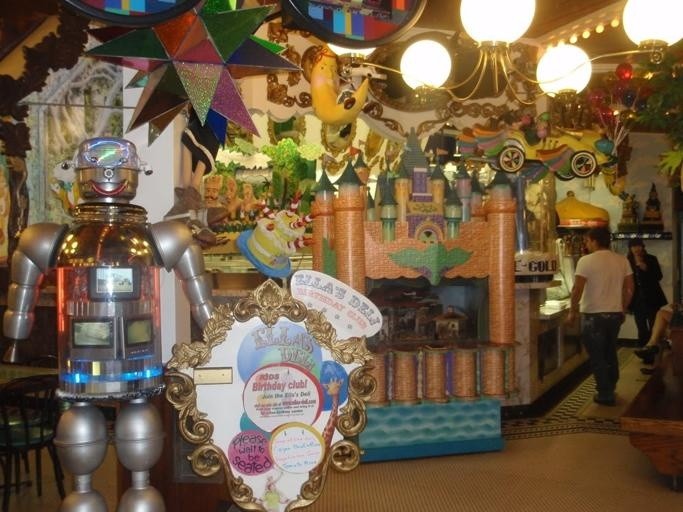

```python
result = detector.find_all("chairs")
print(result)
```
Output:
[0,354,66,511]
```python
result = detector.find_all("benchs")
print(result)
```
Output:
[621,327,683,491]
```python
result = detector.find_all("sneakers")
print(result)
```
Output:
[593,395,615,406]
[634,346,659,357]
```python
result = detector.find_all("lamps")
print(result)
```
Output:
[327,0,682,112]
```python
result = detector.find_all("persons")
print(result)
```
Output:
[571,230,636,405]
[632,303,674,364]
[0,138,212,511]
[626,237,668,347]
